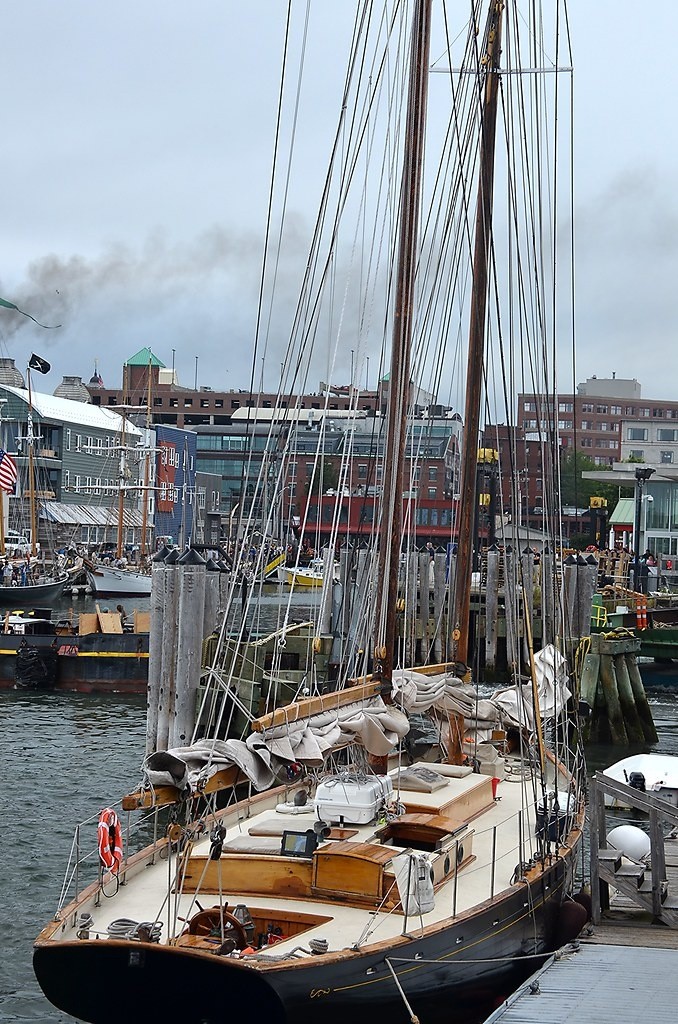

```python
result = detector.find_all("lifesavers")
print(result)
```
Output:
[587,545,597,552]
[97,807,123,872]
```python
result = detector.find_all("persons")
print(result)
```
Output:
[0,538,673,589]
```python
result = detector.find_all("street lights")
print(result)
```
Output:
[634,467,656,591]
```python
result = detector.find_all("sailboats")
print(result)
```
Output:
[0,336,343,599]
[33,0,589,1024]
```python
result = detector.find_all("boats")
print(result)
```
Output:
[1,606,268,696]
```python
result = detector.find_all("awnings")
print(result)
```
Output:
[609,498,636,532]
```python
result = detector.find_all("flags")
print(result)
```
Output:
[0,448,17,496]
